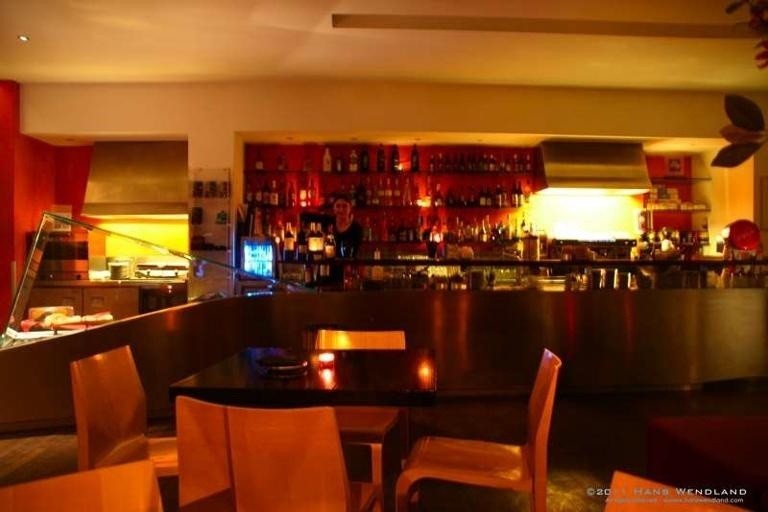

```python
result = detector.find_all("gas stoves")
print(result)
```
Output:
[132,269,188,277]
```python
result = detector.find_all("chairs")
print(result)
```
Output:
[62,341,181,505]
[166,388,389,511]
[390,341,566,511]
[287,322,410,511]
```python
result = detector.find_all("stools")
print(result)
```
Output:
[604,465,752,512]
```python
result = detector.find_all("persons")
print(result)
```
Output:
[301,197,365,283]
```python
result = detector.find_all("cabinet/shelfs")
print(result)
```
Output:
[641,152,712,240]
[189,164,230,264]
[242,139,535,271]
[22,276,185,321]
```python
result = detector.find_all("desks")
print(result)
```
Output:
[0,458,172,512]
[169,344,439,511]
[631,403,767,496]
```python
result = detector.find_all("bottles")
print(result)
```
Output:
[428,153,532,174]
[283,222,295,260]
[320,263,330,285]
[322,142,421,174]
[253,147,310,174]
[303,264,315,290]
[309,222,324,262]
[297,225,307,262]
[325,224,336,259]
[246,179,526,208]
[344,213,765,288]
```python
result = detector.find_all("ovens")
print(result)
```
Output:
[140,286,187,314]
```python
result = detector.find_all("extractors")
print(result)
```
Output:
[79,141,189,221]
